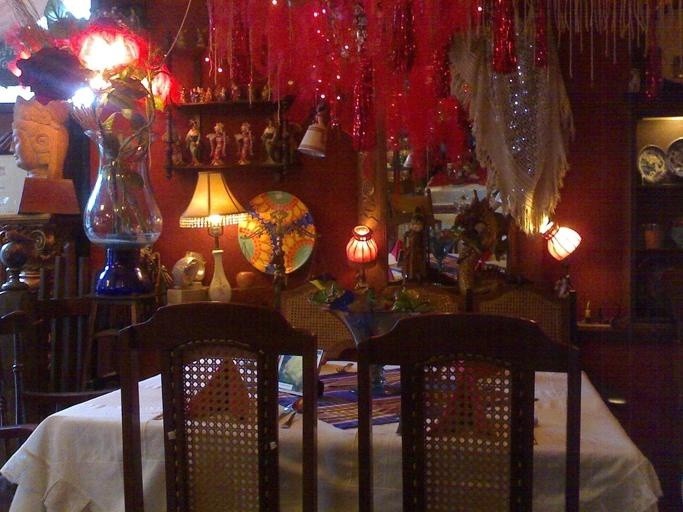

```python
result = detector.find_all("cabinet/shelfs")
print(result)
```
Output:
[165,94,297,181]
[353,95,623,111]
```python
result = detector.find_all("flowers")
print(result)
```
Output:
[10,6,177,110]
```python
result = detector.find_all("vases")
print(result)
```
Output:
[208,249,232,302]
[82,128,164,245]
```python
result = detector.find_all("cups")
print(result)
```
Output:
[639,222,661,249]
[670,219,683,247]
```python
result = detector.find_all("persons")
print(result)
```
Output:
[9,95,70,179]
[165,77,303,170]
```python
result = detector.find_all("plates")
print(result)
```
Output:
[236,190,319,277]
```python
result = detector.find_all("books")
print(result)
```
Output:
[278,350,326,397]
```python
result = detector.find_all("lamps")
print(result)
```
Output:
[298,103,332,157]
[346,225,379,292]
[531,211,578,296]
[179,169,249,249]
